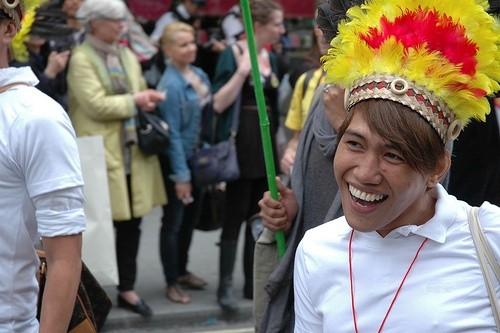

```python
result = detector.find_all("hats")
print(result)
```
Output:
[320,0,500,146]
[0,0,23,33]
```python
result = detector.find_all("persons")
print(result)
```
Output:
[253,0,366,333]
[292,98,500,333]
[0,0,88,333]
[7,0,287,318]
[280,4,333,189]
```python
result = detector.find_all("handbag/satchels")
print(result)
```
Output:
[37,249,113,333]
[134,101,171,154]
[190,138,239,187]
[194,183,227,231]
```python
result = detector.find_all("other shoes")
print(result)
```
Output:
[166,284,190,303]
[179,273,207,289]
[118,293,151,317]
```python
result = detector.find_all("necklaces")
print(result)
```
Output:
[349,228,428,333]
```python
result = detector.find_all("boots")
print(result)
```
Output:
[217,240,240,312]
[244,244,253,299]
